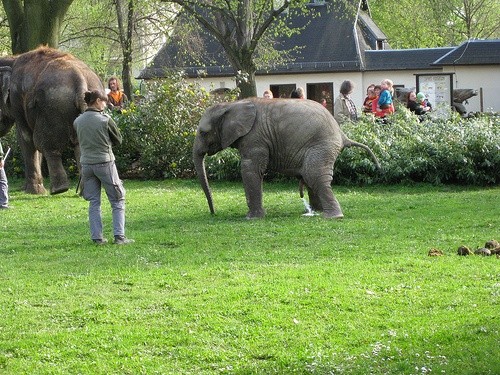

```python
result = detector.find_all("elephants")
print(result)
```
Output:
[190,96,384,224]
[0,43,107,203]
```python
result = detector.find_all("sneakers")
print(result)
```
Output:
[114,235,135,244]
[93,238,108,243]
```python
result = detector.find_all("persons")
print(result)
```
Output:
[318,98,327,107]
[290,88,305,100]
[408,91,431,122]
[378,78,394,124]
[417,93,432,111]
[74,90,135,245]
[107,77,128,113]
[334,81,357,125]
[0,141,13,208]
[372,86,395,125]
[264,91,273,99]
[363,84,379,114]
